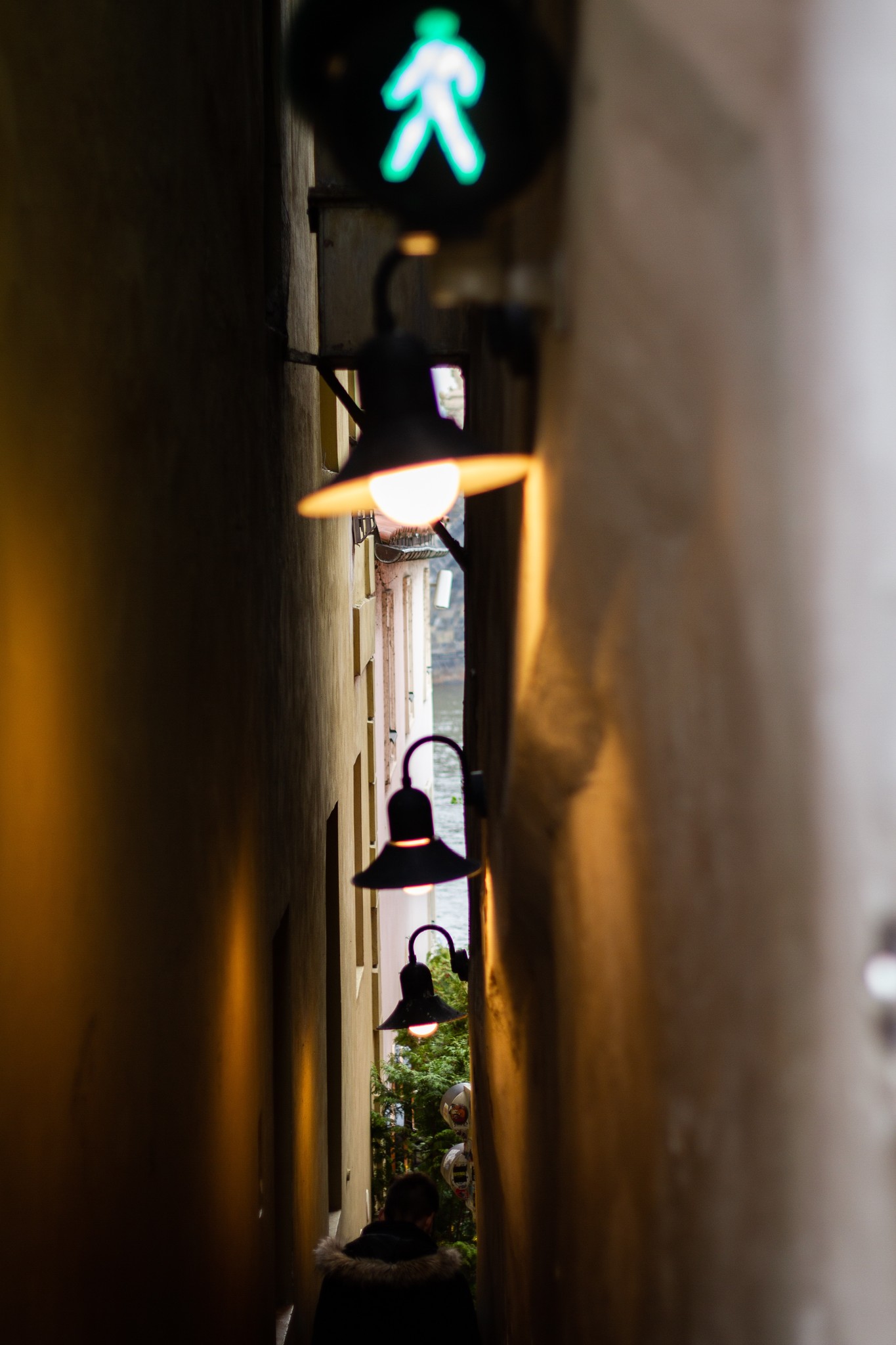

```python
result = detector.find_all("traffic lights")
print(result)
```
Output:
[278,1,571,231]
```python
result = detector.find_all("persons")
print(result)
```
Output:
[313,1172,481,1345]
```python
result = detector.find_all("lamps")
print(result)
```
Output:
[367,732,500,896]
[296,225,545,538]
[370,921,469,1042]
